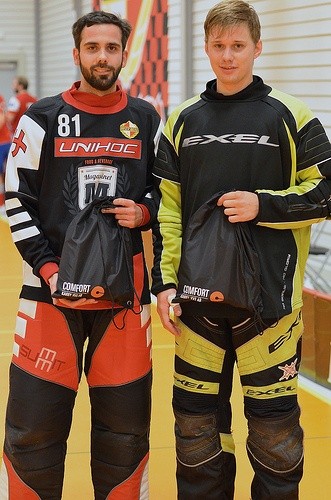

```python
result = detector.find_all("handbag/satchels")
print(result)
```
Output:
[51,196,143,311]
[172,191,264,316]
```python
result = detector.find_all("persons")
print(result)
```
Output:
[0,11,164,500]
[151,0,331,500]
